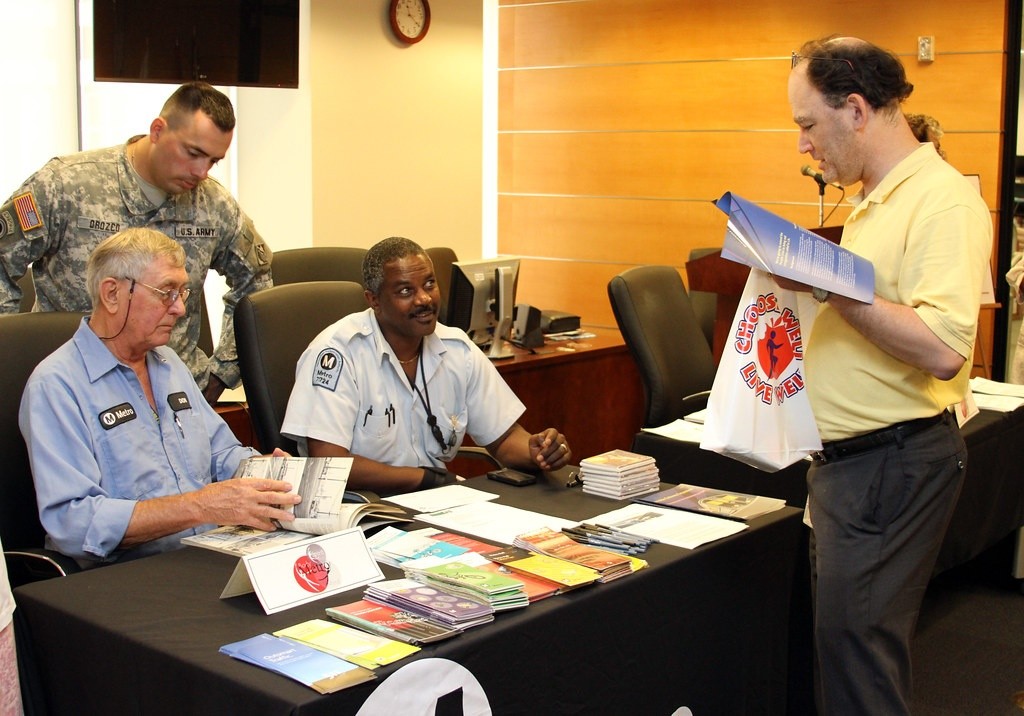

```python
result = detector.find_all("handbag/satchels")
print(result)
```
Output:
[700,265,824,473]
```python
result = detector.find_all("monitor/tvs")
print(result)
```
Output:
[92,0,300,88]
[446,255,520,359]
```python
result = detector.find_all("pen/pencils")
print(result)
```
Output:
[561,523,656,556]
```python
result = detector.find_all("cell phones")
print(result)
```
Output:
[486,468,536,486]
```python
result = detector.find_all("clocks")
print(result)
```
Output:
[390,0,431,43]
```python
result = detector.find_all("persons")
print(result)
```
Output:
[279,236,573,497]
[19,227,301,575]
[788,37,995,716]
[0,80,272,407]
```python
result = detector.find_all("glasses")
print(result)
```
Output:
[791,51,854,73]
[124,274,190,306]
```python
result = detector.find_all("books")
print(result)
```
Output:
[579,449,660,500]
[181,457,414,558]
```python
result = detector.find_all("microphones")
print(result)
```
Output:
[801,165,845,191]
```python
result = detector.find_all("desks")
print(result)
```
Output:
[15,322,1024,716]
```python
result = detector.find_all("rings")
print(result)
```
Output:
[561,444,568,452]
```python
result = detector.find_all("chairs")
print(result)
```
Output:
[606,265,716,430]
[271,248,369,291]
[233,280,369,458]
[0,312,82,592]
[426,247,458,325]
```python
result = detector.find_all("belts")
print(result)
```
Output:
[808,406,952,464]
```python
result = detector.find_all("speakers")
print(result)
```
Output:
[512,303,544,348]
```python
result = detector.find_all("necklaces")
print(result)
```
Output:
[398,352,419,364]
